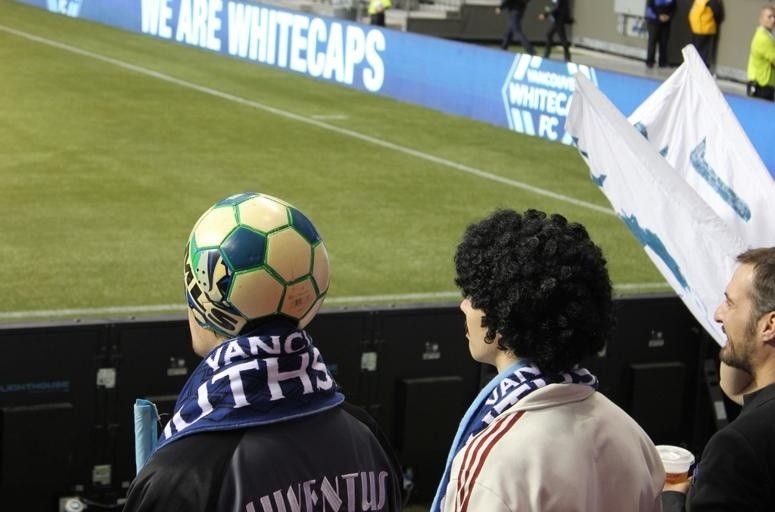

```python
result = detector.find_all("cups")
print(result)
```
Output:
[654,444,695,483]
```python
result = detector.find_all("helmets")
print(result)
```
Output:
[182,189,332,337]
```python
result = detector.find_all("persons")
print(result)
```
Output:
[494,0,775,103]
[428,207,668,511]
[368,0,392,27]
[662,246,775,512]
[124,193,405,512]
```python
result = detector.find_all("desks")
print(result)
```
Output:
[76,471,131,512]
